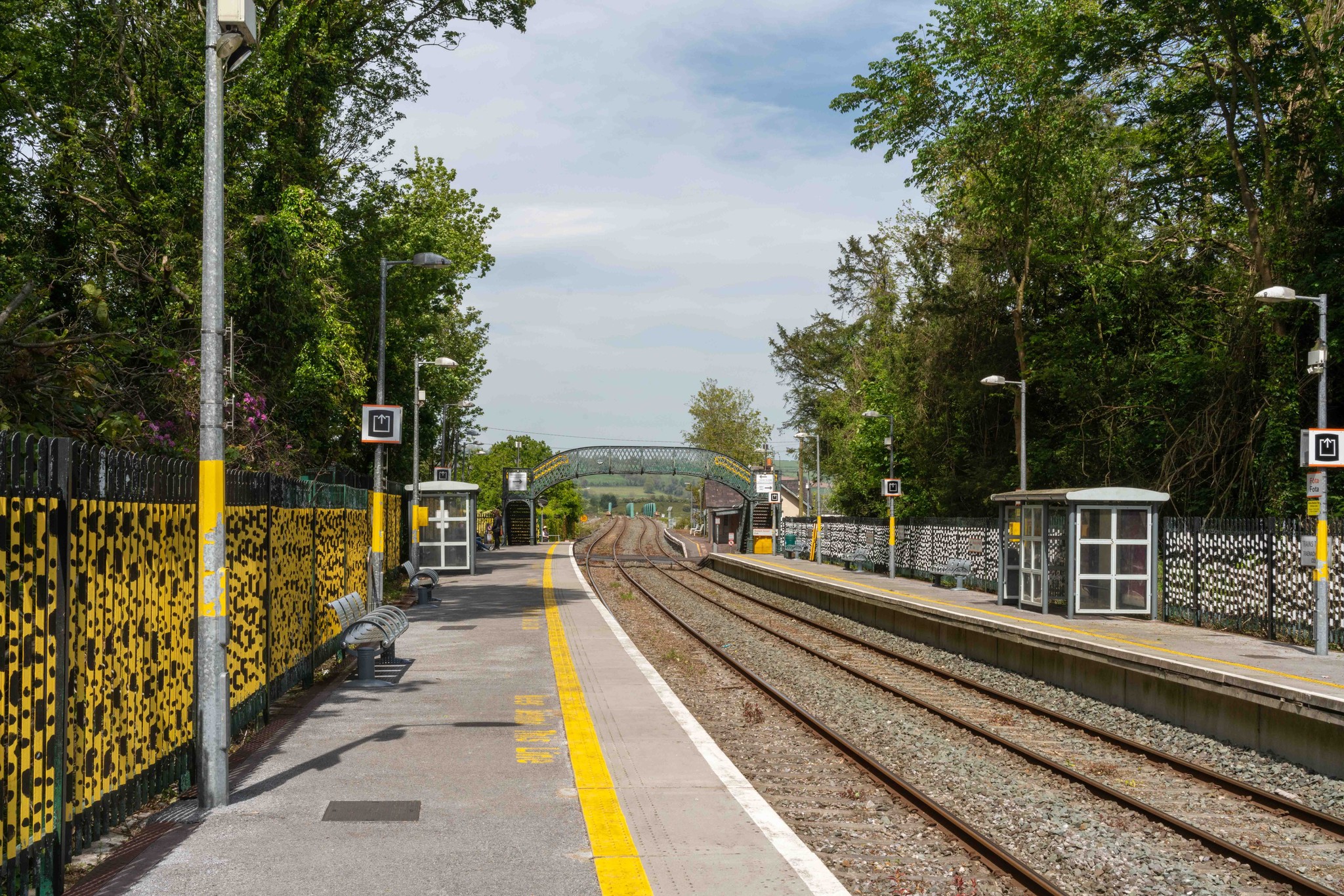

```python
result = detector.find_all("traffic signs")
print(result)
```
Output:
[509,473,527,491]
[756,484,773,493]
[757,474,773,484]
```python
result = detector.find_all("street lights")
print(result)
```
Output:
[684,482,693,529]
[372,252,454,595]
[441,400,475,467]
[462,440,484,483]
[793,432,822,565]
[754,448,776,556]
[467,451,486,482]
[412,356,460,573]
[454,429,482,480]
[1253,285,1330,654]
[861,410,896,579]
[980,374,1026,492]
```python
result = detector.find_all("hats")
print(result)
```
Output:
[543,526,547,530]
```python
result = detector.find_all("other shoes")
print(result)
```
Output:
[478,548,486,551]
[489,544,492,551]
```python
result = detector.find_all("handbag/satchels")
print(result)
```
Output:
[500,529,503,535]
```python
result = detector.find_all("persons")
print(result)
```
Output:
[542,526,549,542]
[491,510,502,551]
[457,522,493,551]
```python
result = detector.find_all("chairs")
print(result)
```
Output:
[690,523,704,537]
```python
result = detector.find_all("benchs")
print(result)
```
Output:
[929,558,972,591]
[326,591,410,689]
[401,561,443,610]
[785,541,804,560]
[841,548,869,572]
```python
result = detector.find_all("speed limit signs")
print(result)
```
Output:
[581,514,587,522]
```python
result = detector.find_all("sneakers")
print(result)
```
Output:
[492,548,500,551]
